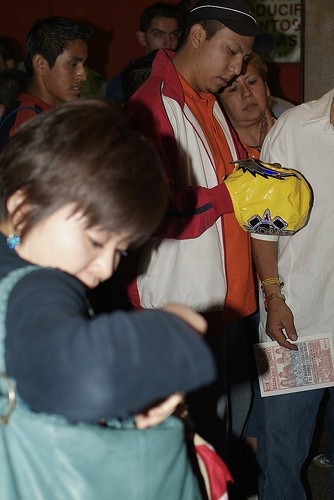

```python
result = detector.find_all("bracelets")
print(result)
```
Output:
[260,278,285,293]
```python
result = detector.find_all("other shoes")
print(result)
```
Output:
[311,453,333,467]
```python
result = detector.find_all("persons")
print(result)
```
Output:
[128,0,259,500]
[0,3,295,455]
[0,98,218,500]
[250,88,334,500]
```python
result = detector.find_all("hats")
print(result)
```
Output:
[190,0,271,50]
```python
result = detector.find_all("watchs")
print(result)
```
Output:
[263,293,286,311]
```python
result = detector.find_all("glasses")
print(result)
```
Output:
[152,30,182,39]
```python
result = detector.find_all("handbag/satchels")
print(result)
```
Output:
[0,265,202,500]
